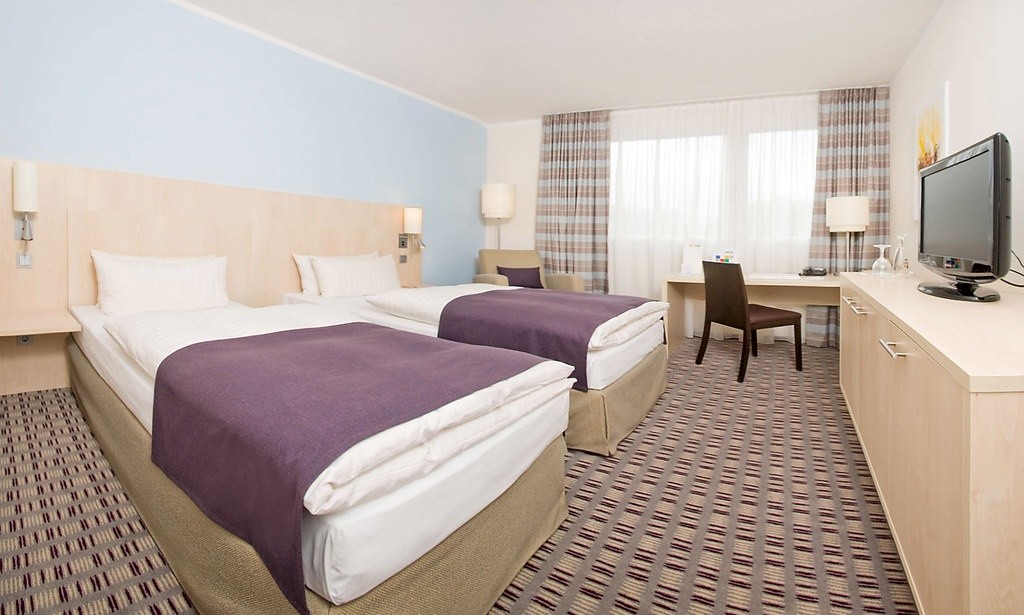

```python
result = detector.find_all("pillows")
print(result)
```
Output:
[313,255,401,295]
[497,266,544,288]
[91,250,232,307]
[293,254,321,296]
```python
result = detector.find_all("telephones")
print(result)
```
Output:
[803,267,827,276]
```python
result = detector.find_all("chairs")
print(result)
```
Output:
[695,261,802,382]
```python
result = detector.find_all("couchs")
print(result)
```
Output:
[473,249,585,293]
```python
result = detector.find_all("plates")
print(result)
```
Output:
[863,269,914,279]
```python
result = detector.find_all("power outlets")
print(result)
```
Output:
[16,335,32,345]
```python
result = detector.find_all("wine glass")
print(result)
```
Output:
[872,244,892,276]
[891,233,910,272]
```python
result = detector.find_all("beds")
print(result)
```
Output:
[68,304,577,615]
[361,283,671,456]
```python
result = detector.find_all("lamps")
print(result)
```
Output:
[482,184,516,249]
[13,161,39,267]
[826,196,870,272]
[403,208,426,254]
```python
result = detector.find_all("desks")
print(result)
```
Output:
[662,274,840,358]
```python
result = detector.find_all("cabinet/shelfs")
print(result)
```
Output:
[840,272,1024,615]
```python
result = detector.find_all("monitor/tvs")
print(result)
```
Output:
[917,131,1013,284]
